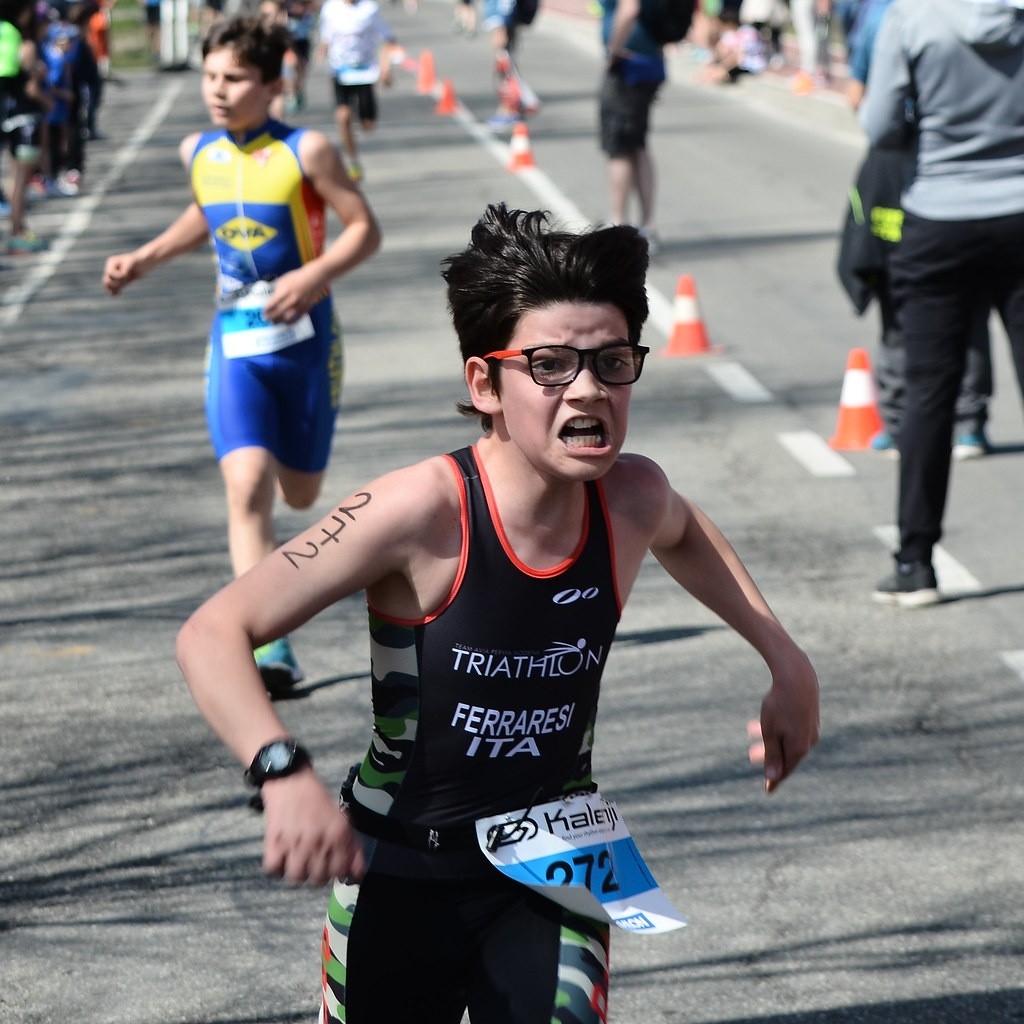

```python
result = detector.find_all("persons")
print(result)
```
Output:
[0,0,112,254]
[101,15,383,696]
[831,0,1024,607]
[702,0,828,96]
[171,201,820,1024]
[311,0,394,181]
[583,0,662,256]
[479,0,523,130]
[254,0,318,121]
[137,0,161,54]
[841,0,993,460]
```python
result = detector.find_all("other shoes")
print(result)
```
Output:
[26,174,48,202]
[10,226,49,253]
[46,176,82,199]
[347,161,362,182]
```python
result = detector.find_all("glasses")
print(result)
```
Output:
[482,345,650,387]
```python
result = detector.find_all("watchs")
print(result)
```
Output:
[243,738,313,791]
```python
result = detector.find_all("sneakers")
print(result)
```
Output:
[253,635,304,693]
[953,433,993,460]
[870,431,900,461]
[871,558,941,607]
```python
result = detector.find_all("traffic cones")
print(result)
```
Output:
[434,82,458,116]
[489,77,537,171]
[413,52,442,97]
[828,346,892,453]
[655,276,724,360]
